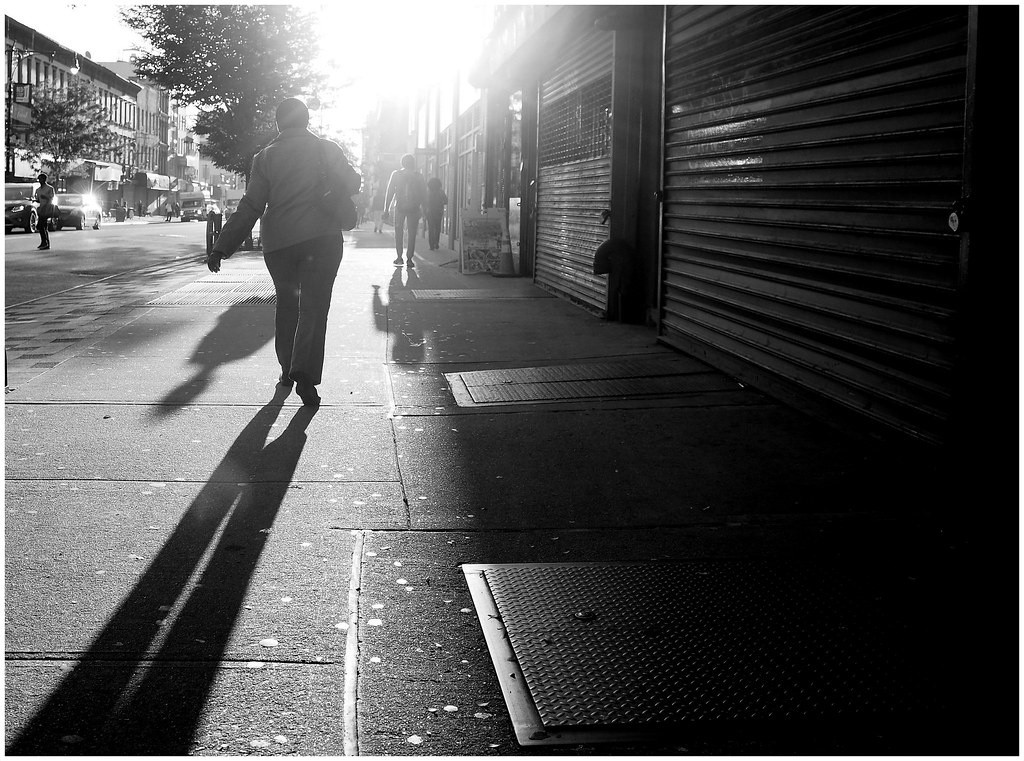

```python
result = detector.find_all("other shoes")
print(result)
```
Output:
[374,227,377,232]
[165,220,168,221]
[406,259,415,267]
[379,230,383,234]
[279,373,294,386]
[37,244,51,250]
[296,380,321,406]
[393,258,404,265]
[428,246,439,250]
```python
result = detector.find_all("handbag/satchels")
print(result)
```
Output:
[313,138,358,231]
[37,204,61,218]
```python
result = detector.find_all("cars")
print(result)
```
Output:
[56,194,103,230]
[5,182,58,233]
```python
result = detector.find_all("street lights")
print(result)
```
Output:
[6,47,81,184]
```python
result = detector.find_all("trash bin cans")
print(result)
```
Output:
[128,207,134,219]
[116,207,126,222]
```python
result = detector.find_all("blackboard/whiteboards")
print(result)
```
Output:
[458,208,508,274]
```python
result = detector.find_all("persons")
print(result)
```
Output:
[34,174,55,250]
[113,200,127,220]
[422,178,448,250]
[138,200,151,217]
[383,154,430,266]
[165,202,179,222]
[207,98,385,407]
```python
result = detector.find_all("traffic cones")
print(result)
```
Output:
[492,228,522,279]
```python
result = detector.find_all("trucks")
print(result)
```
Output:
[179,190,219,222]
[223,189,246,220]
